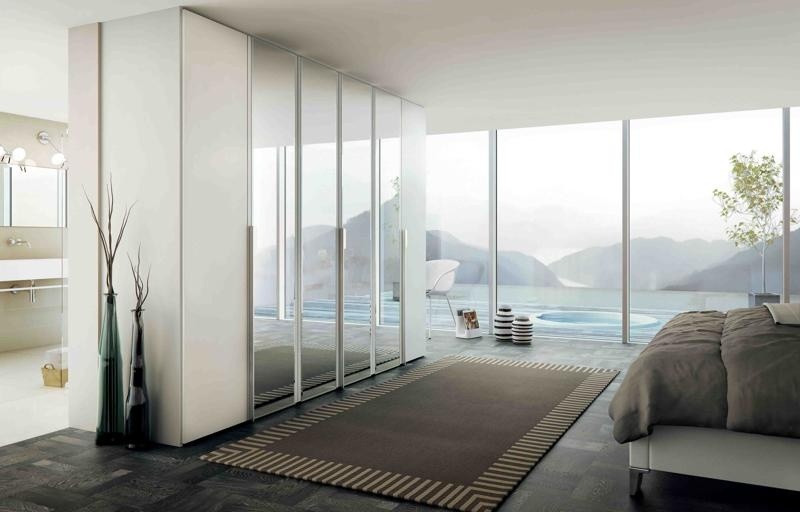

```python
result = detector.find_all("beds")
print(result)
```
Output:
[602,305,800,497]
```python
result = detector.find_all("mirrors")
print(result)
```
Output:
[245,32,410,422]
[2,163,66,227]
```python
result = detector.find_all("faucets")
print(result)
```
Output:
[7,237,32,249]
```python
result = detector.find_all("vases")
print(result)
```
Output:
[96,290,153,452]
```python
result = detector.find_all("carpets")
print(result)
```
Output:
[196,351,622,512]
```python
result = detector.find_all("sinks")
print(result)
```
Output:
[0,258,67,281]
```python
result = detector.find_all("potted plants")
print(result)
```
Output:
[713,150,799,308]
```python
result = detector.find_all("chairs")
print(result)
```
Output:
[419,258,461,340]
